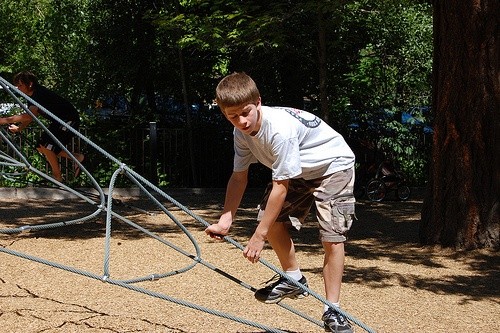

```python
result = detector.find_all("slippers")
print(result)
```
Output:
[74,156,84,179]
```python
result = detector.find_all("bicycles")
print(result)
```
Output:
[364,152,411,203]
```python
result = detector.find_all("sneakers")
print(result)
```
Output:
[255,273,309,304]
[322,308,353,333]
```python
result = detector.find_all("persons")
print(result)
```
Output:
[204,70,356,333]
[0,73,85,183]
